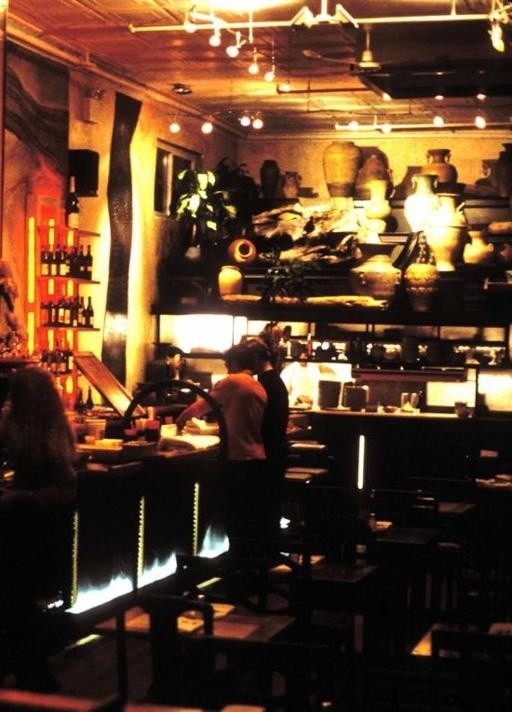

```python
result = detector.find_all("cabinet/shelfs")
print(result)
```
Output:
[37,223,102,377]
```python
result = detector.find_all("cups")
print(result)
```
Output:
[401,392,418,411]
[455,401,467,416]
[402,335,419,364]
[85,419,106,440]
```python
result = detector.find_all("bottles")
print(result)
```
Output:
[219,265,242,295]
[322,141,361,198]
[420,150,457,187]
[39,340,74,376]
[404,235,439,314]
[365,152,394,197]
[39,293,93,328]
[86,388,95,409]
[348,240,404,312]
[282,170,302,197]
[75,388,85,413]
[65,175,80,230]
[39,242,93,281]
[258,158,279,197]
[227,239,256,265]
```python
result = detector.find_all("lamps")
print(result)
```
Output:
[249,46,291,92]
[184,5,246,57]
[336,93,508,133]
[168,108,263,134]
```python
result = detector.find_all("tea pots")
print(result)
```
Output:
[318,379,341,409]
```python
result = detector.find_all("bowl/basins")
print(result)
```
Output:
[475,356,494,364]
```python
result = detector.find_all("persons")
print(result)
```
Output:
[242,338,289,577]
[175,344,267,604]
[0,366,80,602]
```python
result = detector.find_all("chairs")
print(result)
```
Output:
[2,482,512,709]
